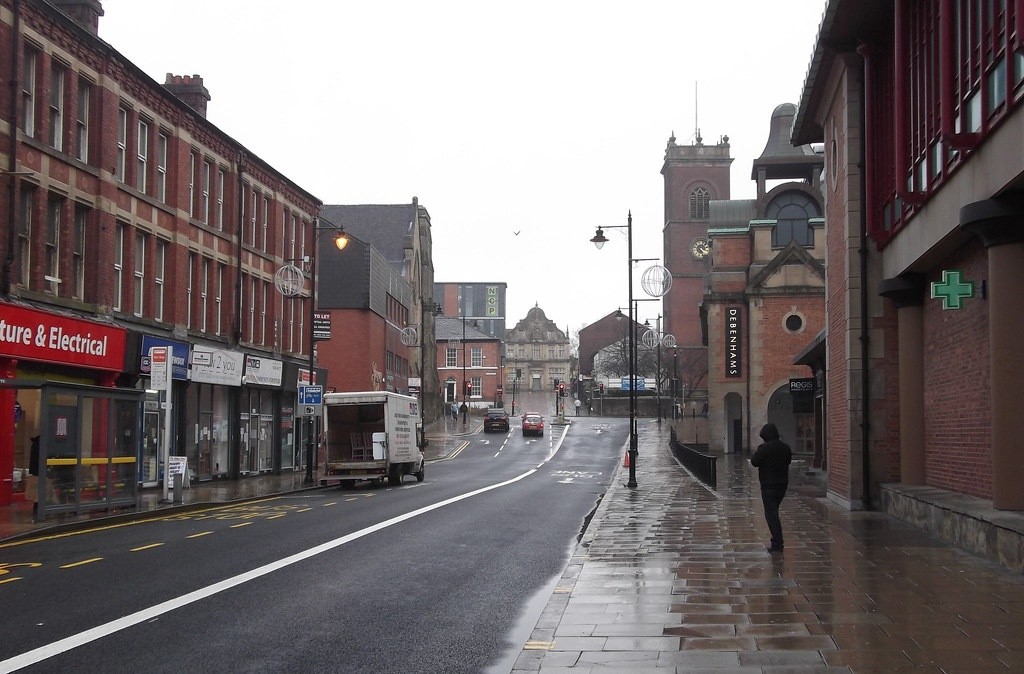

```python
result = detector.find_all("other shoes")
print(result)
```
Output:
[767,538,785,552]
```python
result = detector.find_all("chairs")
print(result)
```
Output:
[363,432,372,459]
[350,433,365,460]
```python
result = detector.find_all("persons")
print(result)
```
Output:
[29,434,39,518]
[702,402,708,415]
[574,399,581,415]
[751,423,792,552]
[585,398,592,415]
[450,401,460,422]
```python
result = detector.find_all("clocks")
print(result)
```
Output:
[692,240,710,257]
